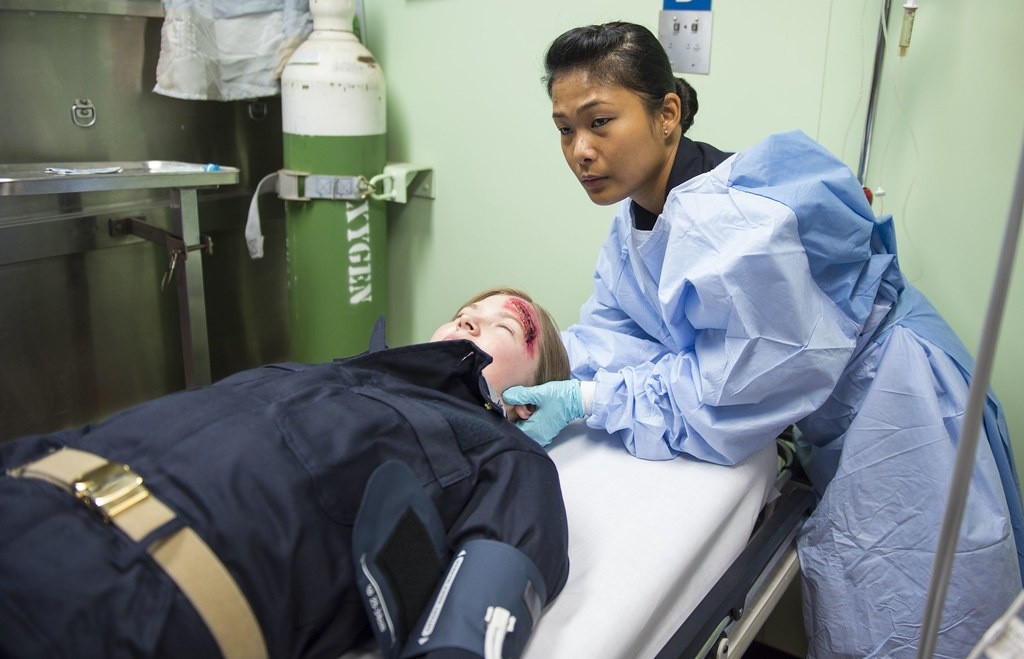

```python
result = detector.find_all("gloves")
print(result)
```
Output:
[502,378,584,446]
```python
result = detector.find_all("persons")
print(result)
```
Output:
[0,287,571,659]
[502,22,1024,659]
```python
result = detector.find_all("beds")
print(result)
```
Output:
[516,417,818,659]
